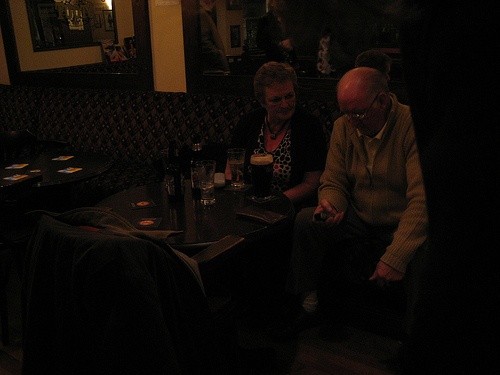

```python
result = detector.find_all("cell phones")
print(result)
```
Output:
[314,211,336,221]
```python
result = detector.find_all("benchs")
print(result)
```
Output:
[0,86,406,340]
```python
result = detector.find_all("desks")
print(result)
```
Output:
[98,185,297,257]
[0,144,110,190]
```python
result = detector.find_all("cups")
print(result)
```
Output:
[251,154,273,201]
[195,160,216,204]
[227,148,245,186]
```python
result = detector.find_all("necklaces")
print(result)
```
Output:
[267,117,287,139]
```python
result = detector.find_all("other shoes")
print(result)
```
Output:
[267,308,324,340]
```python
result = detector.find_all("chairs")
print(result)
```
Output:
[20,208,242,375]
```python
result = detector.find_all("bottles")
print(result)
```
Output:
[190,140,207,192]
[166,150,185,200]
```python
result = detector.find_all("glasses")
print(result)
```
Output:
[335,92,381,121]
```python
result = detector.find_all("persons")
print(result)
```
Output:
[256,0,304,70]
[230,61,328,334]
[267,67,430,342]
[355,51,392,85]
[199,0,232,93]
[318,24,342,74]
